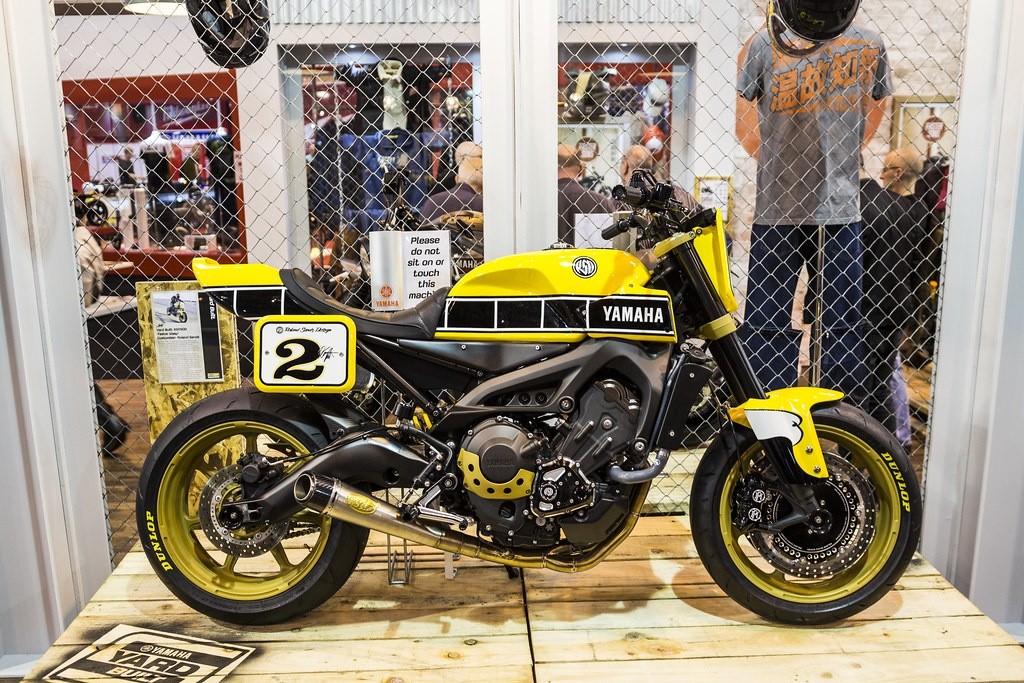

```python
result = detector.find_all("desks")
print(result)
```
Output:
[84,295,138,318]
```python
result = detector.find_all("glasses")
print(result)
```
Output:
[881,166,905,174]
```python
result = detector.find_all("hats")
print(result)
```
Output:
[643,78,669,117]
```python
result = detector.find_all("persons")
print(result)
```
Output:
[880,147,931,343]
[735,25,893,404]
[610,143,699,209]
[557,144,617,245]
[427,141,482,196]
[858,153,915,437]
[76,223,132,456]
[206,126,239,248]
[139,130,185,245]
[118,147,147,184]
[420,156,483,225]
[180,145,202,185]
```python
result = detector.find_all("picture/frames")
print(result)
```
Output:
[889,95,957,160]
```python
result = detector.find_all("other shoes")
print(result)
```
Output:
[102,416,130,456]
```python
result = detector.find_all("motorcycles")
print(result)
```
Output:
[133,164,925,626]
[74,176,119,227]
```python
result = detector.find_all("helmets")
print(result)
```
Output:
[766,0,861,59]
[185,0,271,69]
